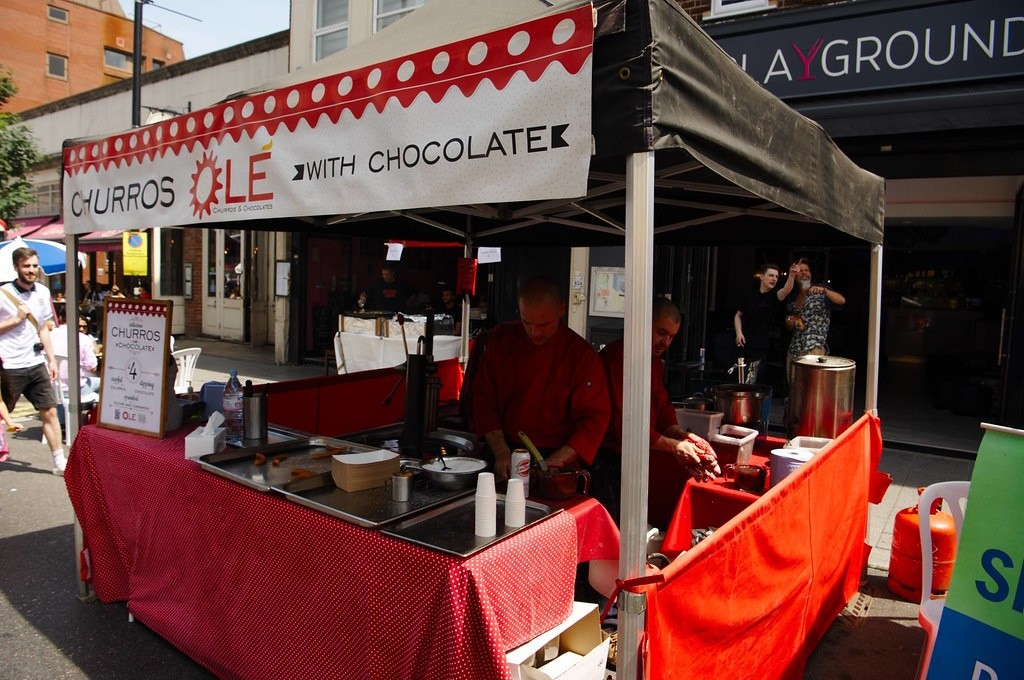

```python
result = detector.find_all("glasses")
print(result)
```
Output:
[78,324,87,329]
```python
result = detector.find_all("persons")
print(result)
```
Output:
[598,298,726,528]
[788,262,846,358]
[358,266,416,313]
[45,303,101,396]
[78,316,99,354]
[0,253,68,476]
[84,280,108,302]
[733,258,801,395]
[467,272,611,482]
[437,289,461,335]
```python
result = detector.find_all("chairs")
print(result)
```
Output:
[40,352,92,447]
[171,347,201,394]
[911,480,971,680]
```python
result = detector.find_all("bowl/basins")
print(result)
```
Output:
[419,456,488,492]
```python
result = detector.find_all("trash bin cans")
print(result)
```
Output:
[758,386,773,436]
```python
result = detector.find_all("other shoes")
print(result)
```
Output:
[52,460,69,476]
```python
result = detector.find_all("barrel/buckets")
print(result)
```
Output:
[710,384,773,448]
[768,448,814,490]
[785,354,857,439]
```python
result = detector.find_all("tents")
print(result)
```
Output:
[45,1,889,680]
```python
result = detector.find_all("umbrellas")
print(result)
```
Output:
[0,236,87,284]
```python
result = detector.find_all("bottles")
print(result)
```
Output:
[222,369,244,443]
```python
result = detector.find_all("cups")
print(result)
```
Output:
[680,397,713,411]
[384,471,413,501]
[537,466,591,502]
[245,393,269,439]
[398,458,424,482]
[504,478,526,528]
[474,472,497,538]
[202,381,228,421]
[723,463,767,493]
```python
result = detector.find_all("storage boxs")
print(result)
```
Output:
[503,600,611,680]
[783,436,833,454]
[707,425,759,466]
[675,406,724,436]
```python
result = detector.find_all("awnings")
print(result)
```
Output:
[0,215,148,252]
